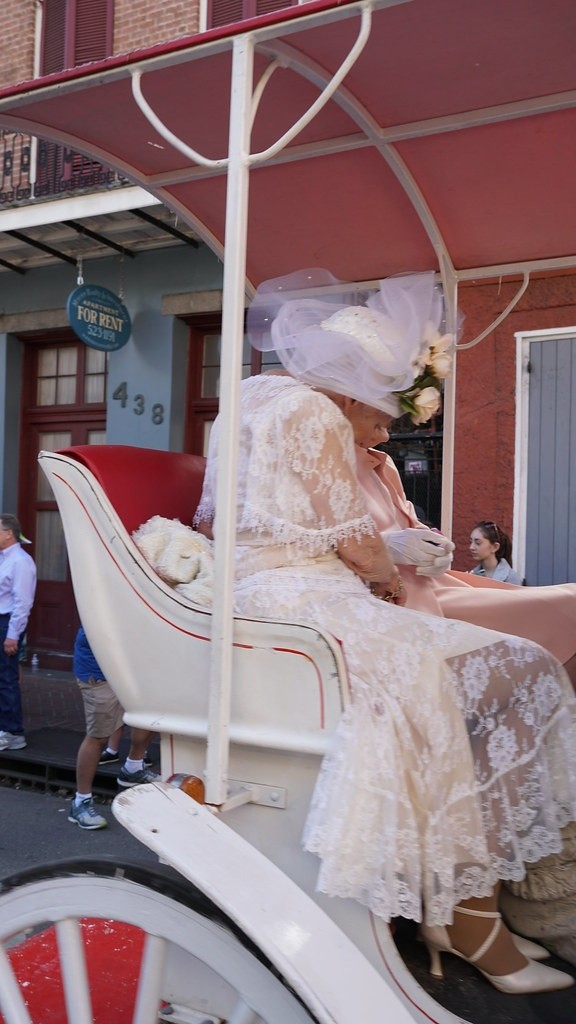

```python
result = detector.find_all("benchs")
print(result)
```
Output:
[38,444,350,753]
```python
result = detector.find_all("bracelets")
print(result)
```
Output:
[372,579,403,601]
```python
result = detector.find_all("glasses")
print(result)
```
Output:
[479,520,500,540]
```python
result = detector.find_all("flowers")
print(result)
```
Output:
[391,333,454,426]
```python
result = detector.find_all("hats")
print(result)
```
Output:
[246,267,465,427]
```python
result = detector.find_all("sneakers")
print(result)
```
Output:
[143,758,152,767]
[0,730,28,751]
[98,751,119,765]
[117,766,161,787]
[68,793,108,830]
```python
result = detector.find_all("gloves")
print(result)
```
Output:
[379,526,449,566]
[415,542,455,578]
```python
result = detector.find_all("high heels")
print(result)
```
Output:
[419,905,575,994]
[509,931,551,961]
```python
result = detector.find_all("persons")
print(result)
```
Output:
[191,305,576,993]
[0,513,38,751]
[465,521,521,587]
[68,625,162,830]
